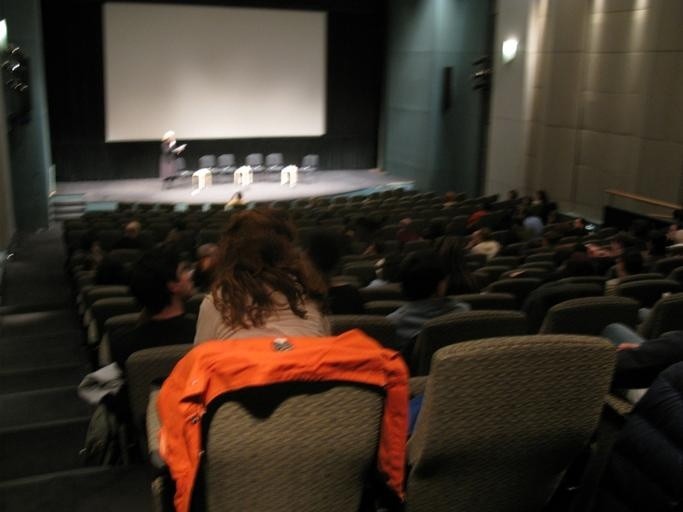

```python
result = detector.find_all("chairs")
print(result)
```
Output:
[177,154,319,187]
[64,189,683,511]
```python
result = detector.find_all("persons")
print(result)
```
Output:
[158,132,181,190]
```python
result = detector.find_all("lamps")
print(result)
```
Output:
[0,40,31,95]
